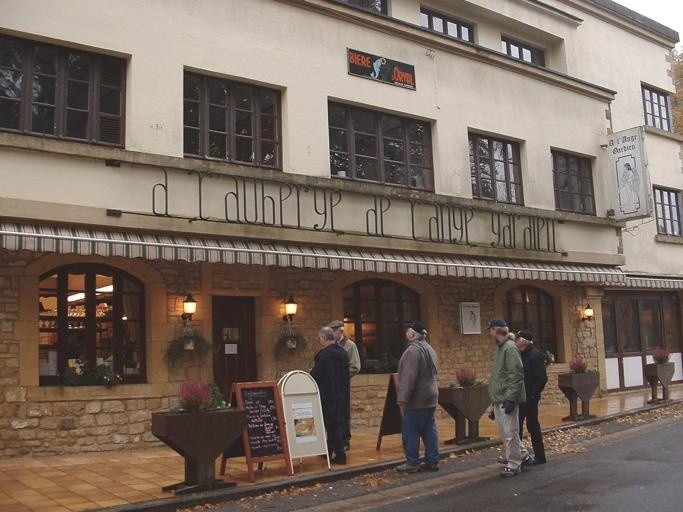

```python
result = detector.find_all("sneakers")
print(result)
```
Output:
[500,465,521,478]
[335,444,350,453]
[330,454,346,465]
[521,452,532,463]
[420,462,439,471]
[396,463,420,473]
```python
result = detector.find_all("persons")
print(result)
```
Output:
[310,326,351,465]
[330,320,362,451]
[396,319,440,473]
[498,329,547,466]
[484,317,534,479]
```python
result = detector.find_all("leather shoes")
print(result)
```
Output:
[529,460,544,464]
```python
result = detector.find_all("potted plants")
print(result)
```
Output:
[163,328,220,368]
[275,335,307,356]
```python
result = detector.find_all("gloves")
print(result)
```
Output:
[501,399,515,414]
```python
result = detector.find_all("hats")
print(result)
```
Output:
[484,319,506,330]
[328,320,344,328]
[403,320,427,334]
[515,330,533,340]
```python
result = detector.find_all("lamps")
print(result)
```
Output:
[182,293,198,320]
[283,297,298,321]
[581,304,594,322]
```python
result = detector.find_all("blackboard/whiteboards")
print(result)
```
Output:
[233,381,289,463]
